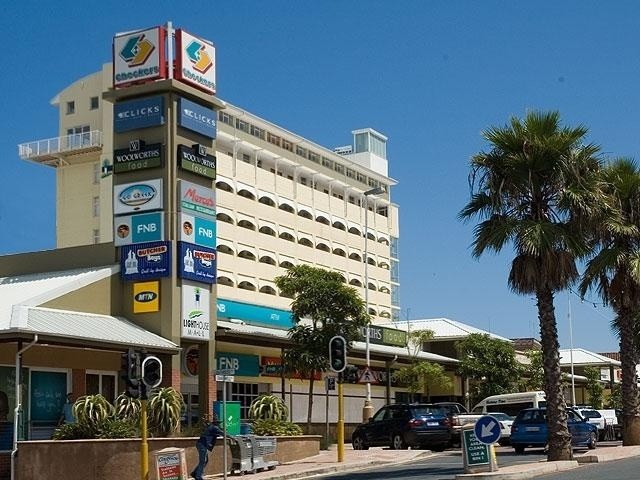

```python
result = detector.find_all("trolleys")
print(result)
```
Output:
[217,429,254,476]
[234,431,281,473]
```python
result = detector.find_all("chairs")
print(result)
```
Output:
[535,414,544,422]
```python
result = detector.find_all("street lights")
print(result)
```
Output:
[362,186,388,421]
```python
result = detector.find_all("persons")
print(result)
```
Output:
[190,417,235,480]
[57,392,79,426]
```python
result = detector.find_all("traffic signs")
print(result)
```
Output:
[213,368,237,376]
[216,375,234,382]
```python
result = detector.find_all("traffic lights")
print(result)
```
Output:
[141,356,163,388]
[124,378,140,399]
[329,336,347,373]
[117,346,136,384]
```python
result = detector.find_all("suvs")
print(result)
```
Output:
[351,389,623,451]
